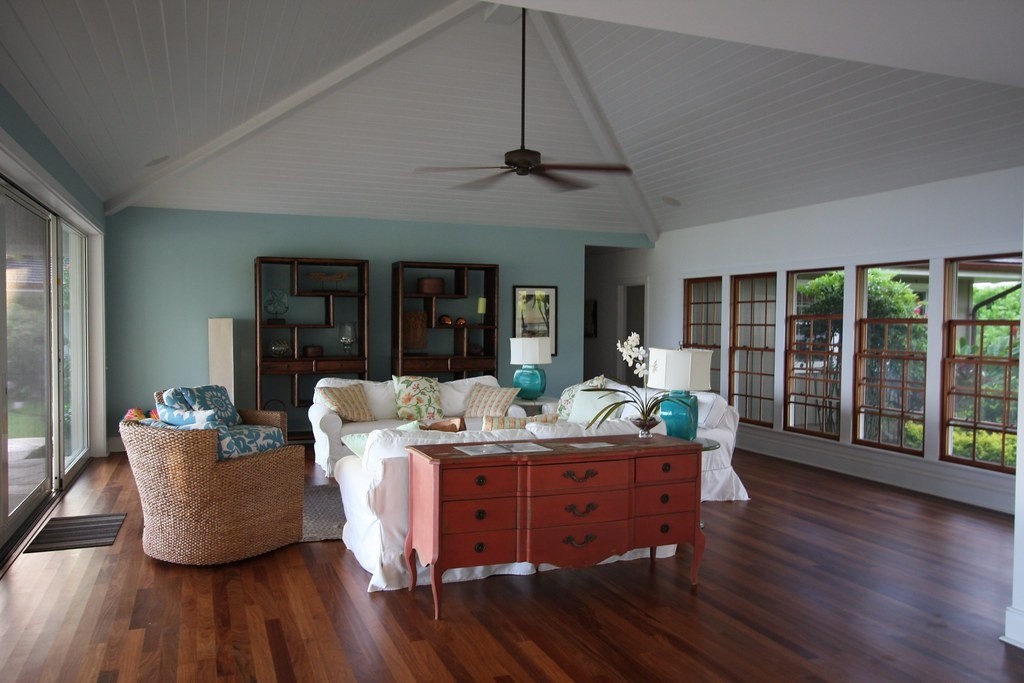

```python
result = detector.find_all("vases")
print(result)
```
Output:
[630,416,662,438]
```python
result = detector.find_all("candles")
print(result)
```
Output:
[478,297,486,314]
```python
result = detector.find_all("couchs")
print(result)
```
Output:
[307,374,751,593]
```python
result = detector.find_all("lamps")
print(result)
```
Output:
[404,311,429,356]
[647,347,714,439]
[510,337,552,400]
[337,323,357,357]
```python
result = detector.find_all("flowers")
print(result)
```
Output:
[581,331,693,430]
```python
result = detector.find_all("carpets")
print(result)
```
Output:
[22,513,128,554]
[300,482,346,542]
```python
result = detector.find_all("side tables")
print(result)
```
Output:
[694,438,720,451]
[514,395,559,416]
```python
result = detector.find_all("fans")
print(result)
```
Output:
[418,8,632,195]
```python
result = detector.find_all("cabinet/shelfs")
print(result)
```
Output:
[391,261,499,382]
[253,257,369,439]
[404,446,706,620]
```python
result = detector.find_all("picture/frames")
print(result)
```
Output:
[513,285,558,356]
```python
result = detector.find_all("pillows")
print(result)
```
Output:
[341,420,420,458]
[156,402,219,426]
[314,383,377,422]
[163,388,192,410]
[392,375,443,420]
[557,375,606,420]
[567,388,625,421]
[180,385,242,426]
[482,414,557,431]
[464,381,521,416]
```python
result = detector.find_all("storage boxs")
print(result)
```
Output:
[208,317,257,413]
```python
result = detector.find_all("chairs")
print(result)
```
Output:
[117,389,305,565]
